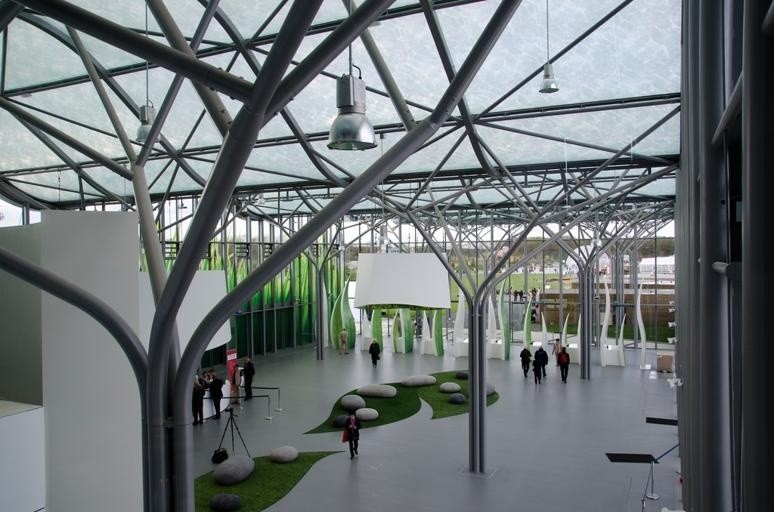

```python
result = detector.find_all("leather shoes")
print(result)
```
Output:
[193,420,203,426]
[231,396,252,405]
[212,414,221,419]
[350,448,358,459]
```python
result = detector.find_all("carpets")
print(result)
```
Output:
[192,448,346,509]
[301,364,499,435]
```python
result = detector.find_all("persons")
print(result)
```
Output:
[344,412,361,460]
[496,287,538,324]
[520,344,531,378]
[551,339,562,367]
[369,339,380,368]
[191,367,224,426]
[533,360,541,384]
[556,347,570,384]
[240,356,255,401]
[339,328,350,355]
[231,364,242,405]
[534,345,548,380]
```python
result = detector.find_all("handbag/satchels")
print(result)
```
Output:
[343,431,348,442]
[377,356,380,360]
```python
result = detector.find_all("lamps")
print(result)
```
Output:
[133,2,161,144]
[536,0,558,96]
[323,0,377,153]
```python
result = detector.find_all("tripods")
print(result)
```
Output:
[218,416,250,457]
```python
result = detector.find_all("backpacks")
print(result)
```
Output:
[560,352,567,365]
